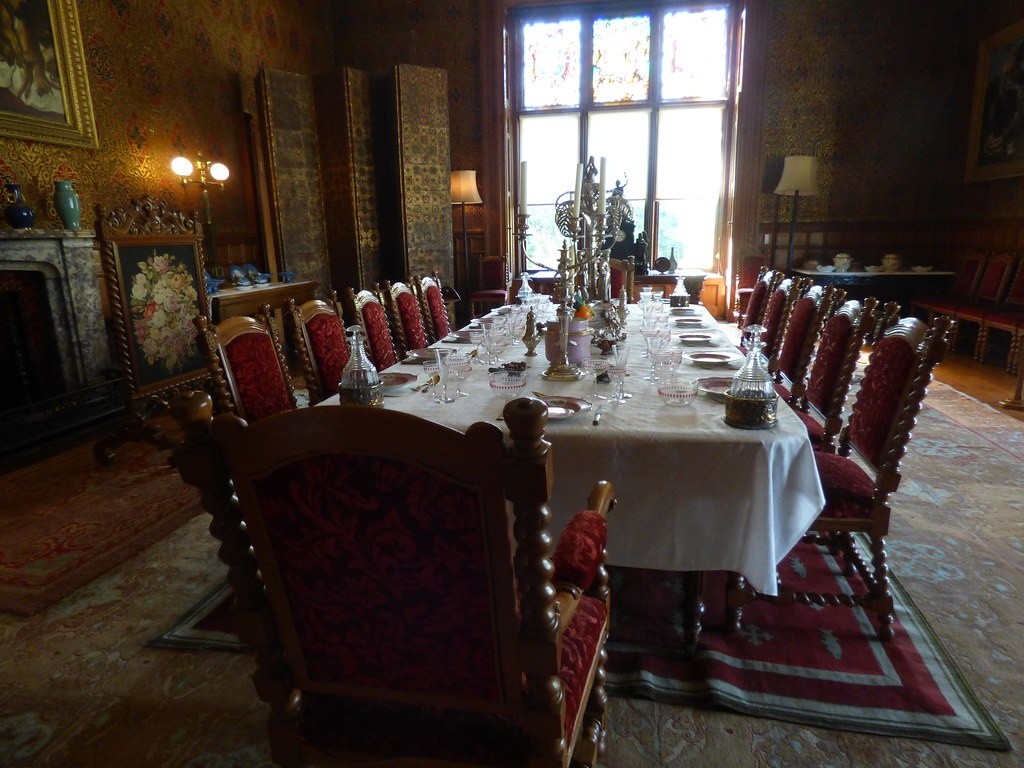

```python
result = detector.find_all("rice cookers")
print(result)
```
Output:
[540,317,593,364]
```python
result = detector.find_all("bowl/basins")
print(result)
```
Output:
[489,372,527,400]
[673,348,683,357]
[671,357,682,369]
[834,253,852,273]
[658,376,699,406]
[882,255,900,271]
[582,358,610,376]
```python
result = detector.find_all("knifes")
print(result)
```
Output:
[592,405,602,424]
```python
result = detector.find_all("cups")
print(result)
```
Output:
[339,380,385,408]
[724,388,779,427]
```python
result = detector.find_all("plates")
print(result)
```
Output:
[866,266,882,272]
[533,396,593,420]
[913,266,932,271]
[672,308,743,401]
[377,373,418,390]
[405,307,511,360]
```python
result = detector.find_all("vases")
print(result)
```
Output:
[53,181,81,230]
[3,183,33,230]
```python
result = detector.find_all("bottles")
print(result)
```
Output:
[668,247,677,274]
[731,324,774,399]
[340,325,379,387]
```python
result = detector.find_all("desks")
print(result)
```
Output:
[212,281,318,363]
[530,270,706,305]
[791,267,956,295]
[312,304,827,653]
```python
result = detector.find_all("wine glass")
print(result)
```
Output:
[611,345,632,398]
[448,356,471,398]
[433,349,455,403]
[637,286,676,388]
[606,362,627,403]
[470,292,552,366]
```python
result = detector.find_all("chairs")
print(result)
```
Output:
[724,265,955,641]
[173,389,618,768]
[910,248,1024,375]
[192,271,452,423]
[468,253,507,319]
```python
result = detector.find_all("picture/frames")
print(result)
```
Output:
[0,0,100,149]
[963,19,1024,185]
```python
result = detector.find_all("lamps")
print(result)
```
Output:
[451,170,483,296]
[773,155,819,278]
[172,148,230,280]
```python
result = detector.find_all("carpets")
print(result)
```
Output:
[0,413,204,616]
[143,531,1014,752]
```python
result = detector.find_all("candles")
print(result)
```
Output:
[596,156,608,214]
[519,161,527,214]
[569,246,575,294]
[573,163,584,217]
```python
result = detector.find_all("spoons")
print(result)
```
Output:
[467,348,479,362]
[422,374,440,392]
